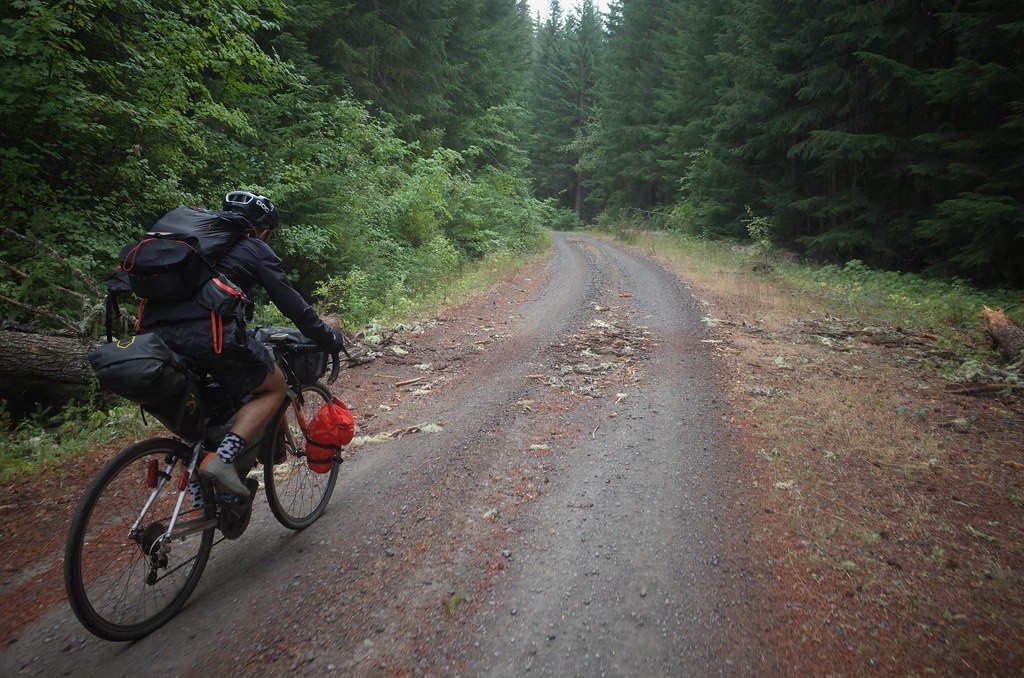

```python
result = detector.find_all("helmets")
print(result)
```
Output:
[222,190,280,230]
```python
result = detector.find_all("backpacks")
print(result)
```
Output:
[118,205,260,304]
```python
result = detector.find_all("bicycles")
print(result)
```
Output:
[61,330,345,642]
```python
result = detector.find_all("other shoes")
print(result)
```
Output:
[197,451,251,498]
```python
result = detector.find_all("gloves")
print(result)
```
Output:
[312,325,343,355]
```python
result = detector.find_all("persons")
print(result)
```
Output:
[139,191,344,497]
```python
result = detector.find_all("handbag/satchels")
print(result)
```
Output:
[306,394,354,473]
[88,332,206,443]
[247,323,330,386]
